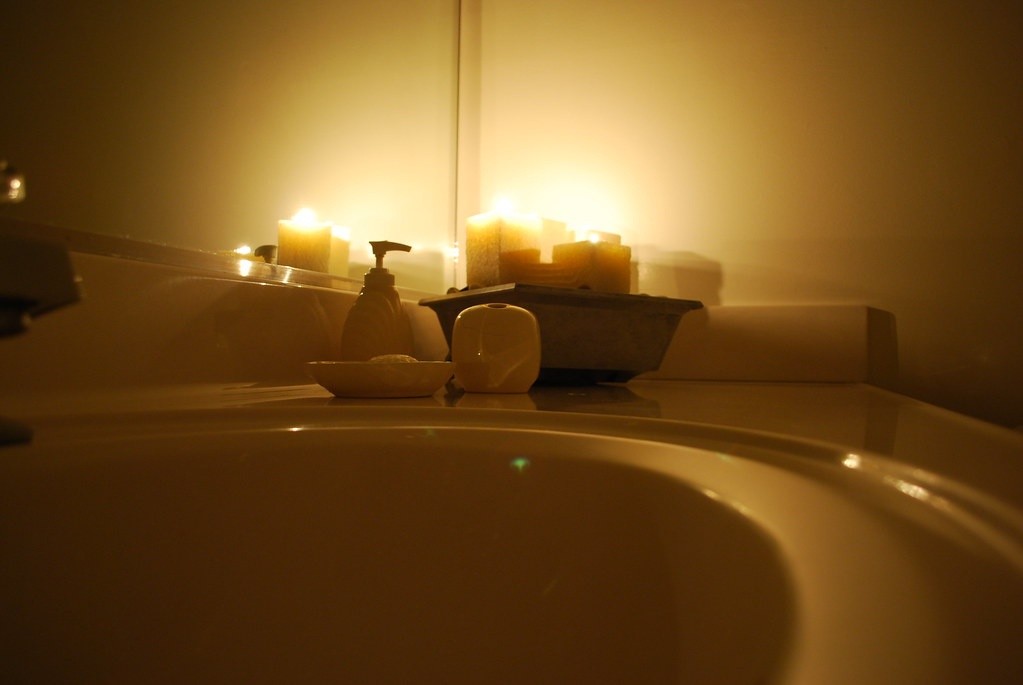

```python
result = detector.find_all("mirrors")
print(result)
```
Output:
[1,0,462,296]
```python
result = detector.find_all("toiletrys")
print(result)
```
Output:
[343,240,412,360]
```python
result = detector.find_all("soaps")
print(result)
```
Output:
[371,355,418,363]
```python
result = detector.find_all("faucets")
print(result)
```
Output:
[0,220,78,340]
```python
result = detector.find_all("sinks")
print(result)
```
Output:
[0,407,1021,685]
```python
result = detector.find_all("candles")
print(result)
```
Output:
[277,208,351,276]
[466,212,631,293]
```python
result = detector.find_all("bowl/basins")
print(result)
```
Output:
[305,361,455,398]
